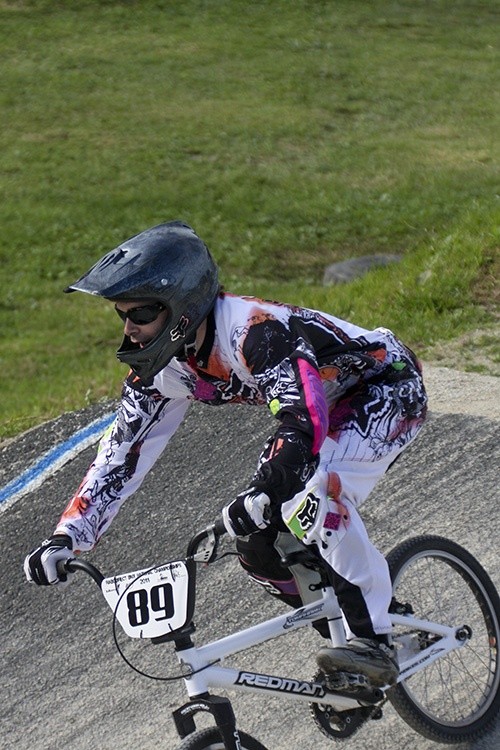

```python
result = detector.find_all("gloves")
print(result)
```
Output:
[24,534,76,586]
[221,486,272,541]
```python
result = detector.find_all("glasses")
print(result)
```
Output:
[114,302,166,325]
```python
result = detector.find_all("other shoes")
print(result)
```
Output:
[318,638,398,687]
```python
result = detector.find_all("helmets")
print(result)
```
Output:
[63,220,221,387]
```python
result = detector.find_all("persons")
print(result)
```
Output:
[24,221,428,686]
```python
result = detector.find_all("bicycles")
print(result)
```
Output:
[54,504,500,750]
